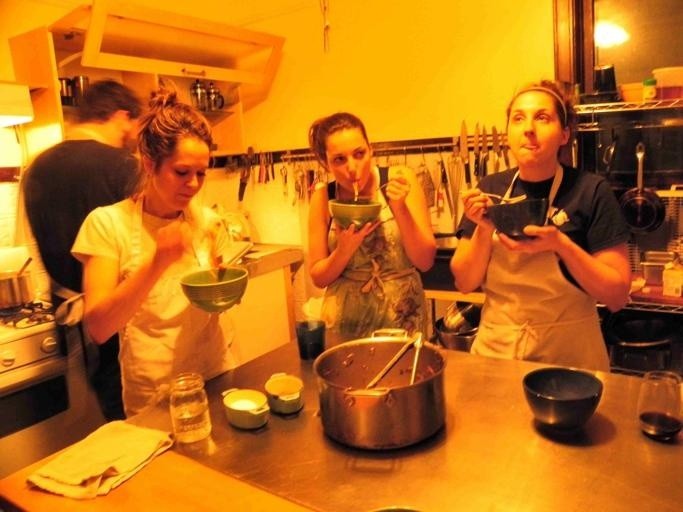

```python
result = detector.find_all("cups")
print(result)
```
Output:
[58,76,90,107]
[637,372,683,440]
[296,323,327,362]
[649,67,682,106]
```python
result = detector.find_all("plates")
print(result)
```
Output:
[577,93,620,103]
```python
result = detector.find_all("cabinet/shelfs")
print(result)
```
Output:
[6,4,283,163]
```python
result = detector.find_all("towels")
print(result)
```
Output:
[23,420,173,501]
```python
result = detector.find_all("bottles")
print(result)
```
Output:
[641,79,657,104]
[189,79,220,112]
[169,372,211,444]
[592,64,618,101]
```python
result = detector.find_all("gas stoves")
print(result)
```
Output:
[0,300,69,396]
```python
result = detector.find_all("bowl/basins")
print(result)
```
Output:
[598,312,674,376]
[484,198,549,241]
[328,198,383,232]
[433,302,478,351]
[179,269,248,314]
[221,387,269,430]
[0,272,35,310]
[265,372,304,416]
[522,367,603,432]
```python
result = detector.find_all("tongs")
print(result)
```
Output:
[365,332,423,386]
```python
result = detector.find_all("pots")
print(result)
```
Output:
[618,142,665,235]
[312,327,445,451]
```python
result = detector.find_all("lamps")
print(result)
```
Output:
[0,82,33,129]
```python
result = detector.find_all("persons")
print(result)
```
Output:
[449,78,634,374]
[69,102,251,420]
[21,78,147,423]
[305,113,436,349]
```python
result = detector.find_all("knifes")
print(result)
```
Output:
[459,120,511,185]
